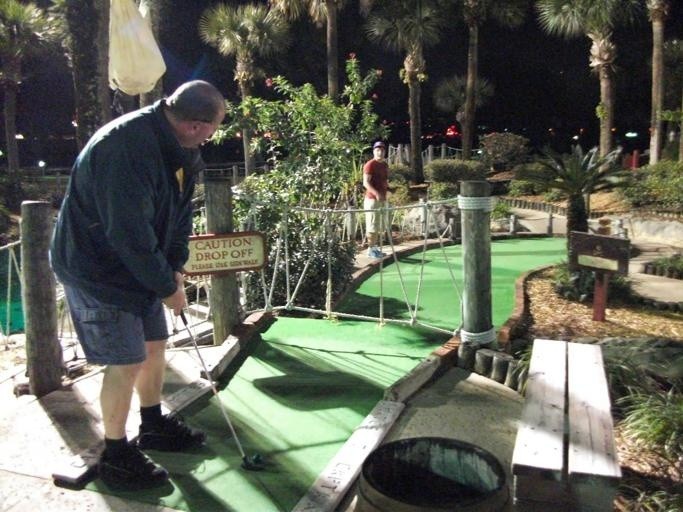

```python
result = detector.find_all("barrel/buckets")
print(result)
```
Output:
[353,436,515,511]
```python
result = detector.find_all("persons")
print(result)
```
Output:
[363,141,390,258]
[49,79,225,493]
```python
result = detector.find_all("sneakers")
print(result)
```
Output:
[366,249,386,259]
[93,443,169,492]
[136,415,206,453]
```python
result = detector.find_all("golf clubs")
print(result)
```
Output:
[181,309,265,471]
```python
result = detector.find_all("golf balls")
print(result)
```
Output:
[252,453,263,464]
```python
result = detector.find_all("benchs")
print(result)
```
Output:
[507,337,622,511]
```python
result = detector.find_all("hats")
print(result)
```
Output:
[372,141,385,148]
